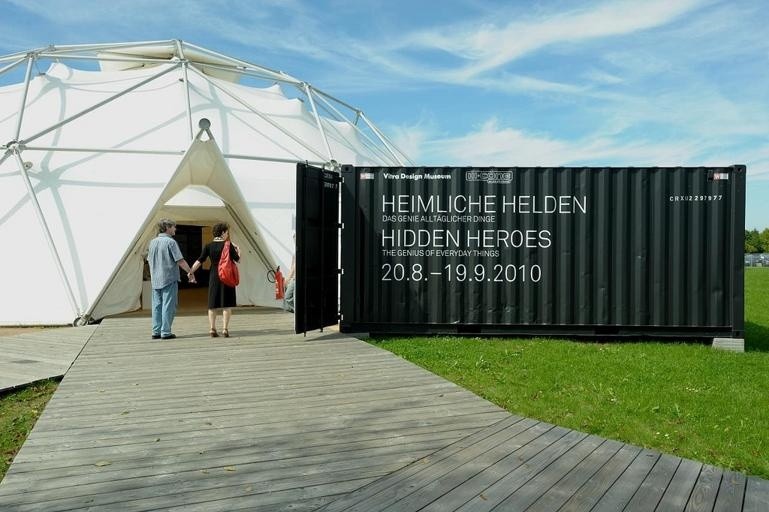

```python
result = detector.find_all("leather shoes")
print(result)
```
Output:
[152,333,177,340]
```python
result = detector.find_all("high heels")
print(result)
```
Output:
[210,328,230,338]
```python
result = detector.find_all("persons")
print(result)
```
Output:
[146,218,197,339]
[185,222,242,338]
[284,252,294,313]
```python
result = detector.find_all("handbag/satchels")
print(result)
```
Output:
[217,239,240,288]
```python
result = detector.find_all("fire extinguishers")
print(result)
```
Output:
[267,267,285,300]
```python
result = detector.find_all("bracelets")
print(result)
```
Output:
[189,272,192,275]
